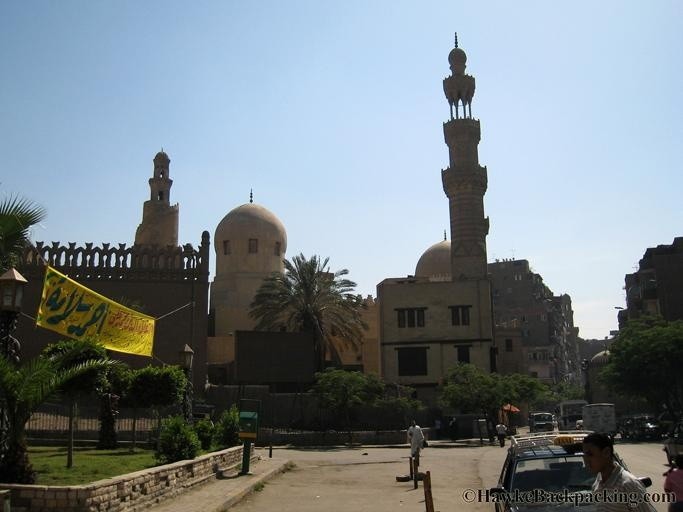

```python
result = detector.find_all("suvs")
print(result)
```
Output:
[489,400,683,512]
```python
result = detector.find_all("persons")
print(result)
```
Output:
[496,422,507,447]
[664,452,683,512]
[406,419,425,466]
[552,418,560,433]
[581,431,652,512]
[447,416,457,442]
[433,417,441,440]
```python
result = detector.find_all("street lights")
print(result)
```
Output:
[0,266,28,482]
[177,342,193,425]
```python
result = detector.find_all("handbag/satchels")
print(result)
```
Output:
[422,439,428,448]
